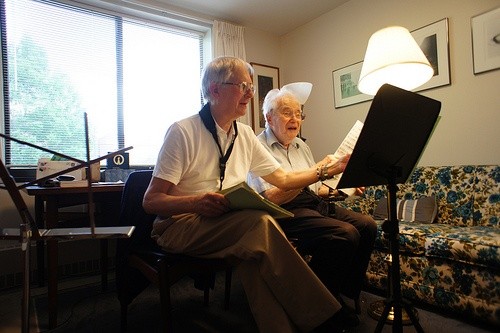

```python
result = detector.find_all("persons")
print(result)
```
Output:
[143,55,350,333]
[247,82,377,327]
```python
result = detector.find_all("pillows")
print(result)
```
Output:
[373,196,438,222]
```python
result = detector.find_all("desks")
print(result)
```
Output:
[21,181,125,329]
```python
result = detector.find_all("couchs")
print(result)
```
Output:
[302,165,500,325]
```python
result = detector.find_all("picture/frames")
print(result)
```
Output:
[332,60,375,109]
[409,17,452,93]
[470,6,500,74]
[249,62,281,136]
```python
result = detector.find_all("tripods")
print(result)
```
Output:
[335,84,442,333]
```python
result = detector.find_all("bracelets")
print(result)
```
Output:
[316,164,333,181]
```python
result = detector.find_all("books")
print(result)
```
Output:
[215,180,294,218]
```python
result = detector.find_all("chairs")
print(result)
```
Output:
[114,170,233,333]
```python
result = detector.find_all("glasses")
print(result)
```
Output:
[221,82,255,95]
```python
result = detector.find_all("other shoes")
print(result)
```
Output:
[337,298,359,325]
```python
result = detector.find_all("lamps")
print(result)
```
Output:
[356,25,434,325]
